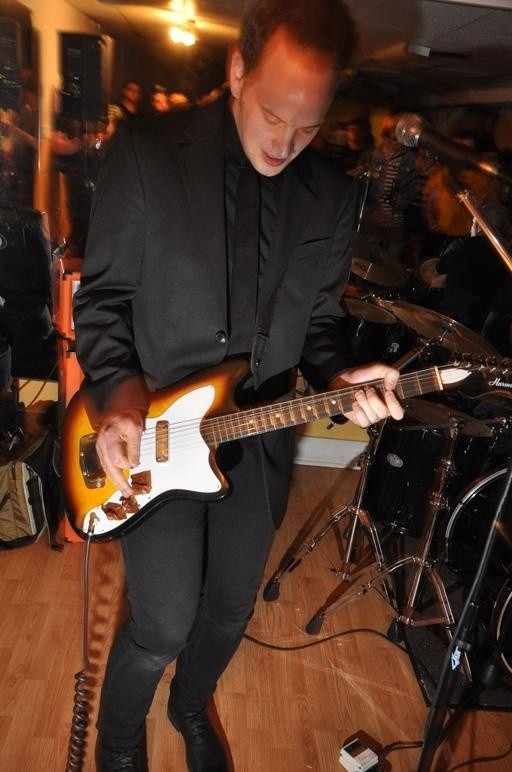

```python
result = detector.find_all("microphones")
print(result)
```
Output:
[395,113,503,177]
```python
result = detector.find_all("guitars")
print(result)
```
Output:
[59,352,512,541]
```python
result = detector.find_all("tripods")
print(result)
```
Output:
[263,419,404,644]
[305,432,484,708]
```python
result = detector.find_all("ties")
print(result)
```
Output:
[228,156,260,356]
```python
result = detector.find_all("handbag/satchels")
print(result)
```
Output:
[0,400,60,551]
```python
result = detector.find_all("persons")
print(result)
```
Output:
[76,0,357,772]
[1,76,512,389]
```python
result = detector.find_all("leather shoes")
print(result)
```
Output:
[167,706,225,772]
[100,741,141,771]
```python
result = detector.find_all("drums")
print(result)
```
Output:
[433,442,511,570]
[362,395,496,538]
[340,295,397,324]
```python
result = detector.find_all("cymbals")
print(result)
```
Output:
[349,238,406,287]
[374,300,511,357]
[398,397,492,440]
